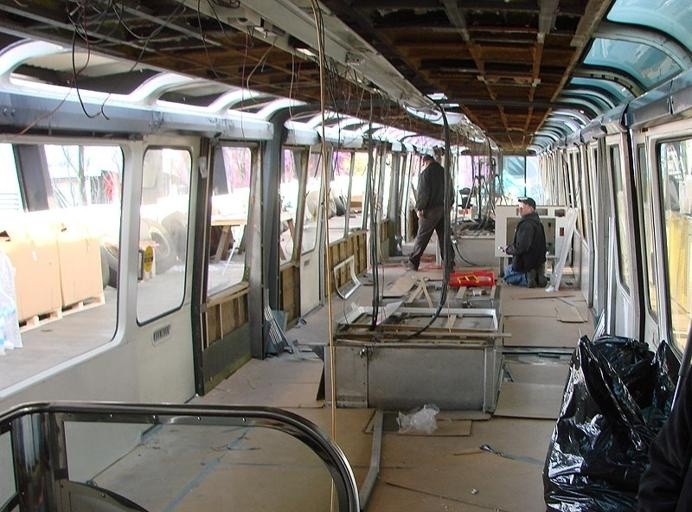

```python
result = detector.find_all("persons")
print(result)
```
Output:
[400,154,457,273]
[501,196,548,288]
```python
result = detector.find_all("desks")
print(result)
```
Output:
[212,206,304,270]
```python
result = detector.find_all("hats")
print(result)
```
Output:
[422,155,432,165]
[519,198,536,208]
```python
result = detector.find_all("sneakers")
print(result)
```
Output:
[401,260,417,270]
[527,270,536,288]
[538,268,546,287]
